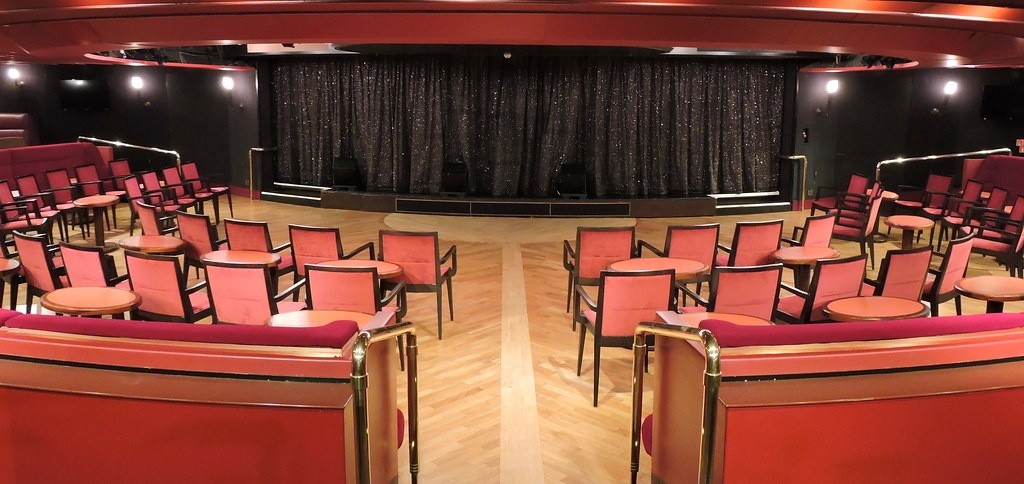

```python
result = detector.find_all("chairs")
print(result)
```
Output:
[561,172,1024,408]
[0,159,461,374]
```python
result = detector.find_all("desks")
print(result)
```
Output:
[960,190,991,199]
[267,311,375,331]
[198,249,282,268]
[953,275,1024,314]
[867,190,899,243]
[73,195,121,254]
[768,246,839,292]
[607,257,710,315]
[1003,206,1013,213]
[0,258,21,277]
[680,311,774,326]
[885,215,935,250]
[317,260,404,279]
[118,235,189,253]
[40,286,142,318]
[821,295,931,321]
